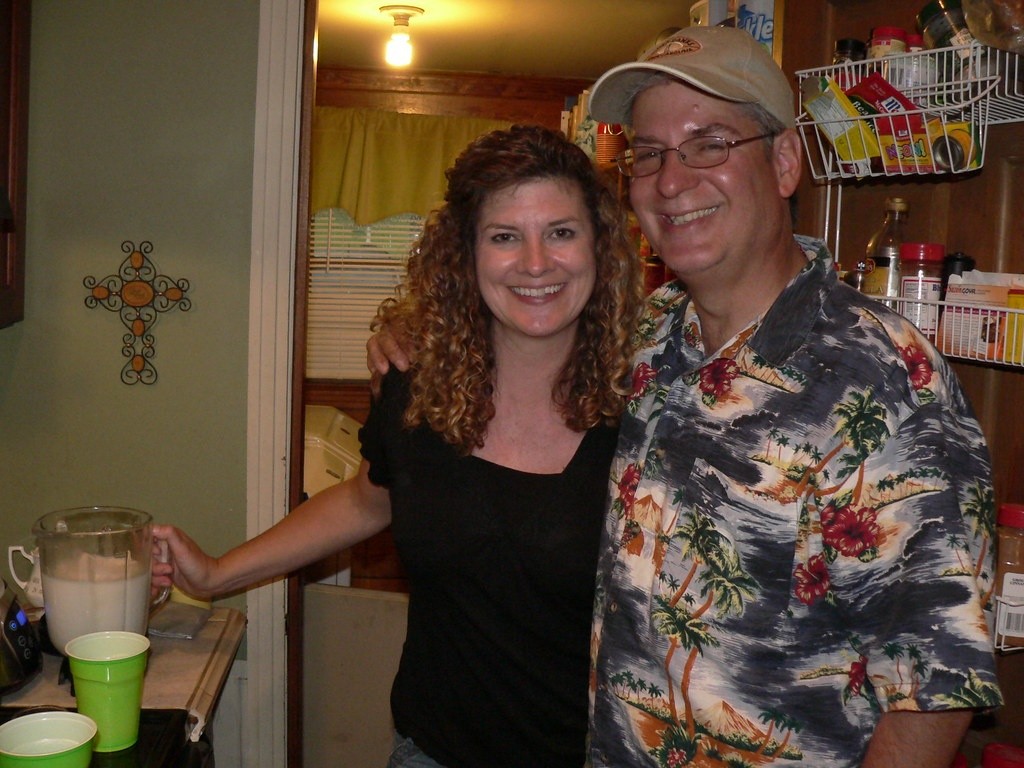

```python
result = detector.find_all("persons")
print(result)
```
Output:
[364,29,1008,768]
[128,121,645,768]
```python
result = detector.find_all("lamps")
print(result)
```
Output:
[378,3,424,68]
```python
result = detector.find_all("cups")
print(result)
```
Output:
[62,630,152,753]
[1,713,97,766]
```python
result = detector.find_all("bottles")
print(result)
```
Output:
[862,199,906,313]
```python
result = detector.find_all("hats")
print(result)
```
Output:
[588,26,795,131]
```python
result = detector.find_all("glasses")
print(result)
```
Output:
[613,129,783,178]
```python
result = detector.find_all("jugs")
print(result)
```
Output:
[8,544,46,609]
[32,506,171,661]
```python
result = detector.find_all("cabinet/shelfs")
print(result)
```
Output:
[791,40,1023,653]
